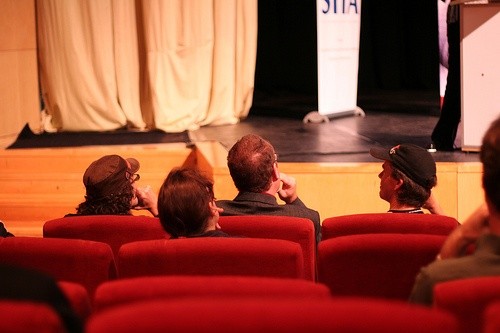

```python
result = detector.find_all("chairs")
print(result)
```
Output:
[0,213,500,333]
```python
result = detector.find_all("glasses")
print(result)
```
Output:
[130,173,140,185]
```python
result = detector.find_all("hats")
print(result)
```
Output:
[82,154,140,199]
[180,144,213,186]
[369,143,436,188]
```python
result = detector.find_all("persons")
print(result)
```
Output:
[157,167,232,239]
[410,117,500,304]
[214,133,321,242]
[376,142,441,216]
[418,0,460,153]
[66,154,158,218]
